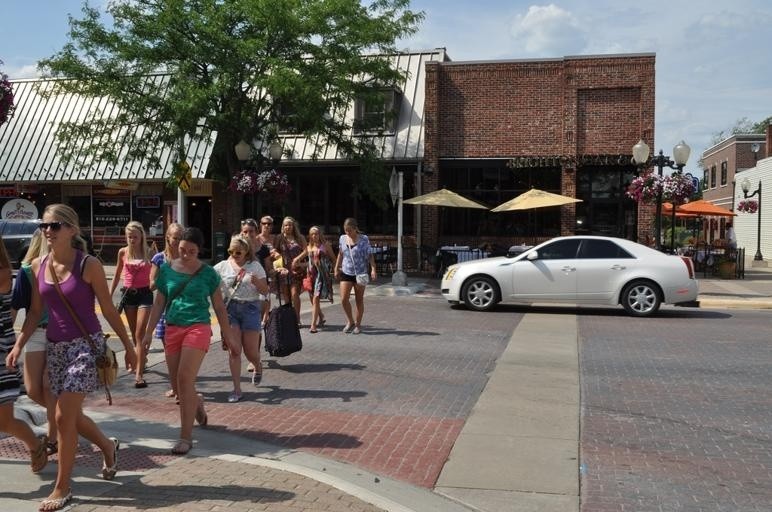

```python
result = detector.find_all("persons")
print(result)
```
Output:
[9,228,59,455]
[0,237,49,472]
[142,227,241,456]
[724,222,737,258]
[6,203,139,512]
[110,219,157,389]
[148,222,186,405]
[213,214,377,401]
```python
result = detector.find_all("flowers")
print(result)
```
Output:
[226,169,287,196]
[736,200,757,214]
[625,173,697,206]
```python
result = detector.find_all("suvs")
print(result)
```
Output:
[0,218,43,269]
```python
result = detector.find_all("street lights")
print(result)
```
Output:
[234,139,285,225]
[741,177,762,260]
[630,136,692,255]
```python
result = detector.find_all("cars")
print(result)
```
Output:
[440,232,701,317]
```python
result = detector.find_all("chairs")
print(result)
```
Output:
[372,242,507,278]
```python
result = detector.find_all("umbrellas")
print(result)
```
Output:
[489,184,584,246]
[666,199,737,271]
[402,188,489,244]
[651,202,703,244]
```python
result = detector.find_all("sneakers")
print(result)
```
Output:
[252,362,261,386]
[228,392,243,403]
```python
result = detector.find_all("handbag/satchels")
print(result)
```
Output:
[116,296,127,313]
[355,274,369,287]
[303,277,313,291]
[95,344,118,385]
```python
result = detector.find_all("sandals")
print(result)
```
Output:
[171,438,193,454]
[102,437,119,480]
[38,486,72,511]
[30,434,58,472]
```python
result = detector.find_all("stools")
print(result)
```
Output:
[93,249,104,262]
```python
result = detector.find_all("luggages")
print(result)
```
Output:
[264,270,303,356]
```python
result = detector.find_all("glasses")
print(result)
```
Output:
[39,221,66,232]
[228,249,243,256]
[241,220,252,225]
